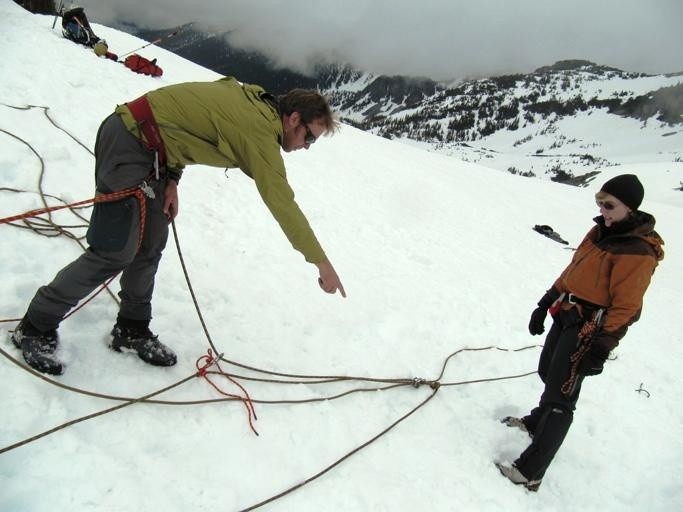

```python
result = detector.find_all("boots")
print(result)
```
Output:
[106,314,178,367]
[11,315,66,376]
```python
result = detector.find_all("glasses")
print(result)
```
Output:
[598,201,621,210]
[300,119,316,144]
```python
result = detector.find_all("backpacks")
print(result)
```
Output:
[62,8,109,55]
[124,54,163,79]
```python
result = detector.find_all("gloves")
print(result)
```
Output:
[574,340,610,376]
[529,306,549,336]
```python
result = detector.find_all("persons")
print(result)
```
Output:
[489,172,669,492]
[9,74,352,377]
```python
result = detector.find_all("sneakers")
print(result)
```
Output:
[501,415,536,439]
[494,462,543,492]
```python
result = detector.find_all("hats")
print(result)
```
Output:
[601,174,645,211]
